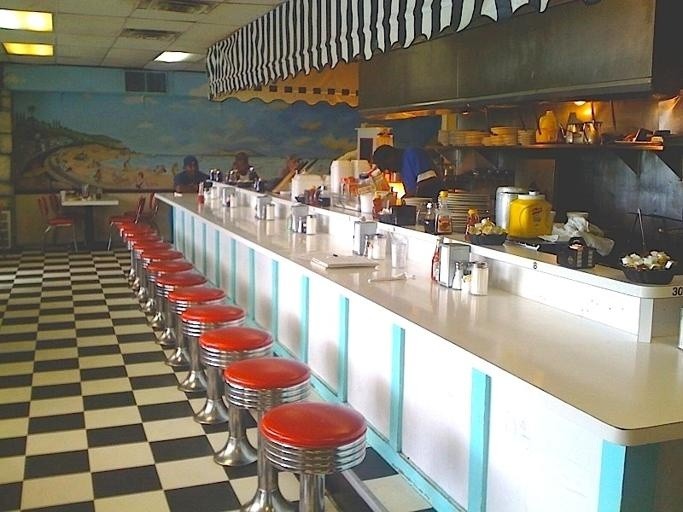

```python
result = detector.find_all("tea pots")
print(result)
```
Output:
[583,119,601,145]
[559,122,584,144]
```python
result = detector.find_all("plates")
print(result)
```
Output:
[438,189,492,235]
[401,197,433,225]
[437,127,535,146]
[614,139,663,144]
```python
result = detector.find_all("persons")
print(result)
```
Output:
[169,155,211,194]
[224,152,265,193]
[373,143,442,199]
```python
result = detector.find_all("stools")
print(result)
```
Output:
[199,327,274,466]
[261,404,368,512]
[224,357,312,511]
[118,223,244,422]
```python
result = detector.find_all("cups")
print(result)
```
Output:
[195,195,204,203]
[65,184,102,201]
[391,239,406,267]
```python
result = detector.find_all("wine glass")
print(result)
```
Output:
[598,128,606,145]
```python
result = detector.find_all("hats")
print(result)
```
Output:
[287,154,302,161]
[183,155,198,168]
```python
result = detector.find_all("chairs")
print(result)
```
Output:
[125,192,159,235]
[50,194,88,247]
[38,196,79,254]
[107,198,145,251]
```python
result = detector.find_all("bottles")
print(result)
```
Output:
[287,214,316,234]
[254,204,275,220]
[372,186,406,221]
[381,168,396,182]
[226,193,238,208]
[352,234,387,259]
[434,191,454,235]
[464,208,479,240]
[430,237,488,295]
[424,203,434,233]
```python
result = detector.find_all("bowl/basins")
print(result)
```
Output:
[468,234,507,245]
[618,264,678,285]
